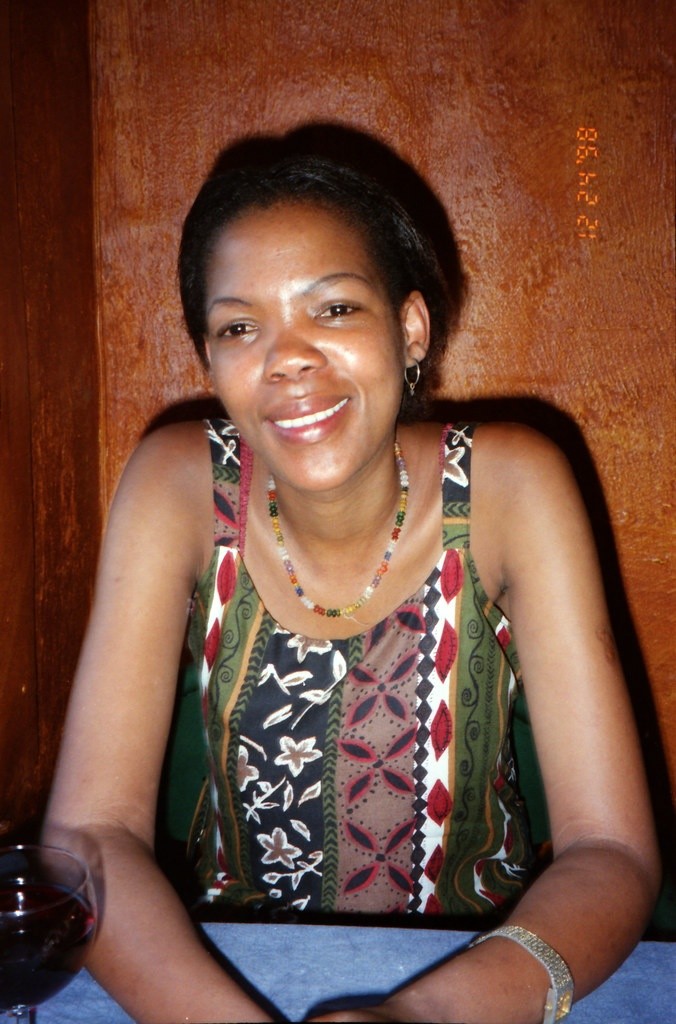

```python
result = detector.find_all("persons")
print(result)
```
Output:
[35,125,666,1022]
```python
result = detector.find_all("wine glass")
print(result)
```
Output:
[0,843,97,1024]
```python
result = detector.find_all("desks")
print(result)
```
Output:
[1,922,676,1024]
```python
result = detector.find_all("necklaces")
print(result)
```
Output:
[267,435,411,619]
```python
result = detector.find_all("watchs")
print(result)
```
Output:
[469,925,576,1024]
